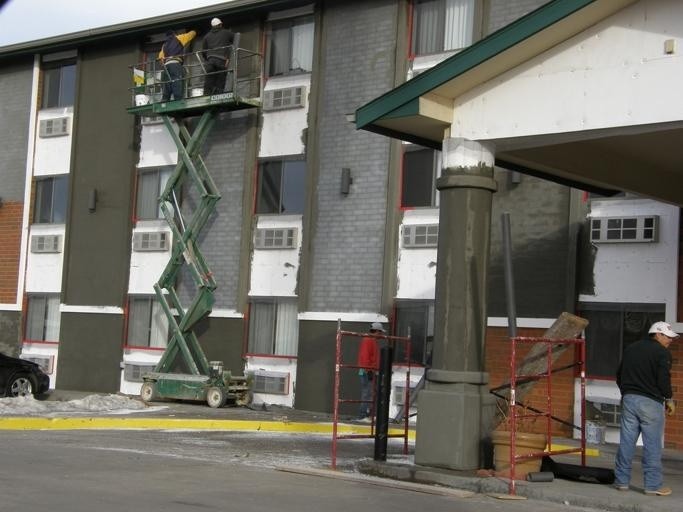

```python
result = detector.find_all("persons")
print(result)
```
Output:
[354,321,387,424]
[201,17,236,98]
[157,28,197,102]
[613,319,681,496]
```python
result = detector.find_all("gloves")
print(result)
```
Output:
[367,370,373,381]
[664,399,675,416]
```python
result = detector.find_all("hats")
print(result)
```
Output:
[370,322,386,333]
[647,320,680,340]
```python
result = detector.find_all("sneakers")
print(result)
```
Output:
[359,416,372,424]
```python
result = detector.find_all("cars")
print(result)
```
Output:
[0,352,49,400]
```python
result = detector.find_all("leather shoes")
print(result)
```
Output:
[612,483,628,491]
[644,487,672,496]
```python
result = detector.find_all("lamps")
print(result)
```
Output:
[342,167,353,196]
[88,188,98,211]
